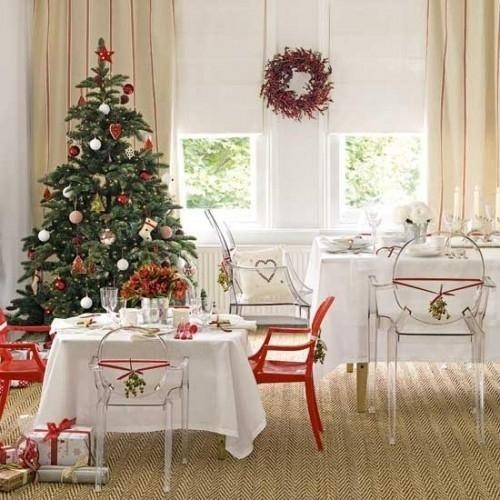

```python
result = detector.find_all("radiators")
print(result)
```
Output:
[191,244,314,318]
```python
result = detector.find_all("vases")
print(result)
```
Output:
[402,223,427,244]
[142,297,169,327]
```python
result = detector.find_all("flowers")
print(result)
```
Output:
[395,200,435,226]
[260,44,334,121]
[119,263,189,305]
[183,262,198,289]
[218,261,231,292]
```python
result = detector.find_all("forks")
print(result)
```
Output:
[174,323,185,339]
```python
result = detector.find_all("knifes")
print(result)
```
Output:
[181,323,192,339]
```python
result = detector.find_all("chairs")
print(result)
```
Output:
[366,227,497,446]
[204,207,313,331]
[0,307,51,424]
[248,294,336,451]
[88,325,189,493]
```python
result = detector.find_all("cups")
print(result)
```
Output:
[428,236,445,252]
[115,298,138,327]
[173,297,213,327]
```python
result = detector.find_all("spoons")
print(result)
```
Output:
[188,325,198,339]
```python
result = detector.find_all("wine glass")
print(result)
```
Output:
[100,286,117,323]
[366,204,382,253]
